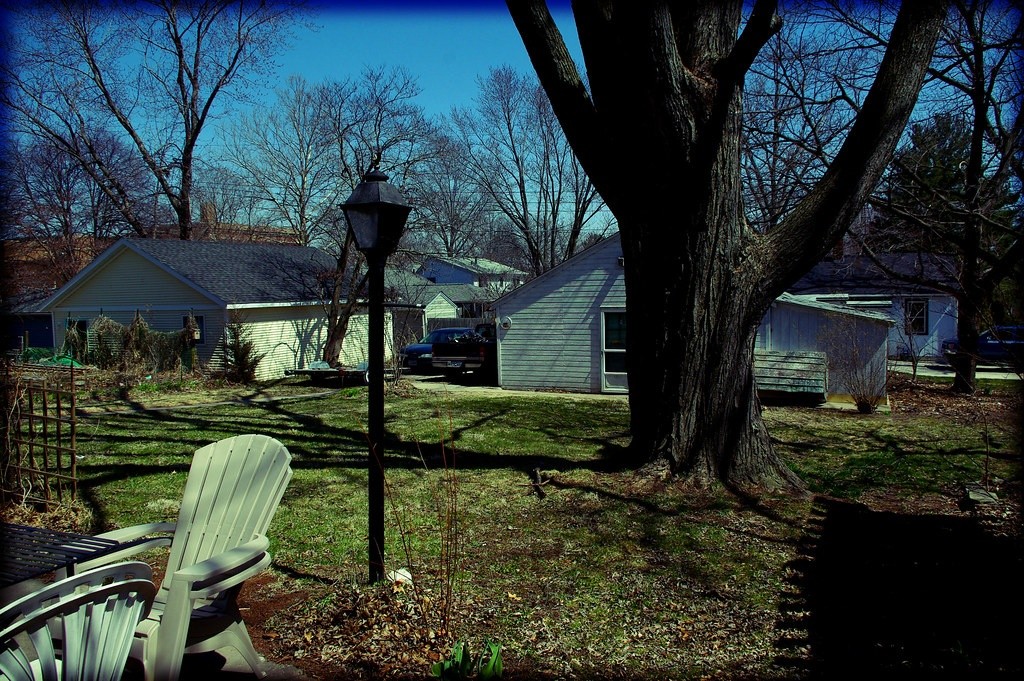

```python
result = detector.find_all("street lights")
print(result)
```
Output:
[336,165,415,585]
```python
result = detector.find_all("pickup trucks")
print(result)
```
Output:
[433,323,497,384]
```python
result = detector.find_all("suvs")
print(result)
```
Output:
[399,327,482,374]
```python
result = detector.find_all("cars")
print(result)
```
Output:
[941,326,1024,367]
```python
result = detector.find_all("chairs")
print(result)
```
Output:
[0,561,157,681]
[55,433,293,681]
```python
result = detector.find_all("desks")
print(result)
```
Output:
[0,520,119,589]
